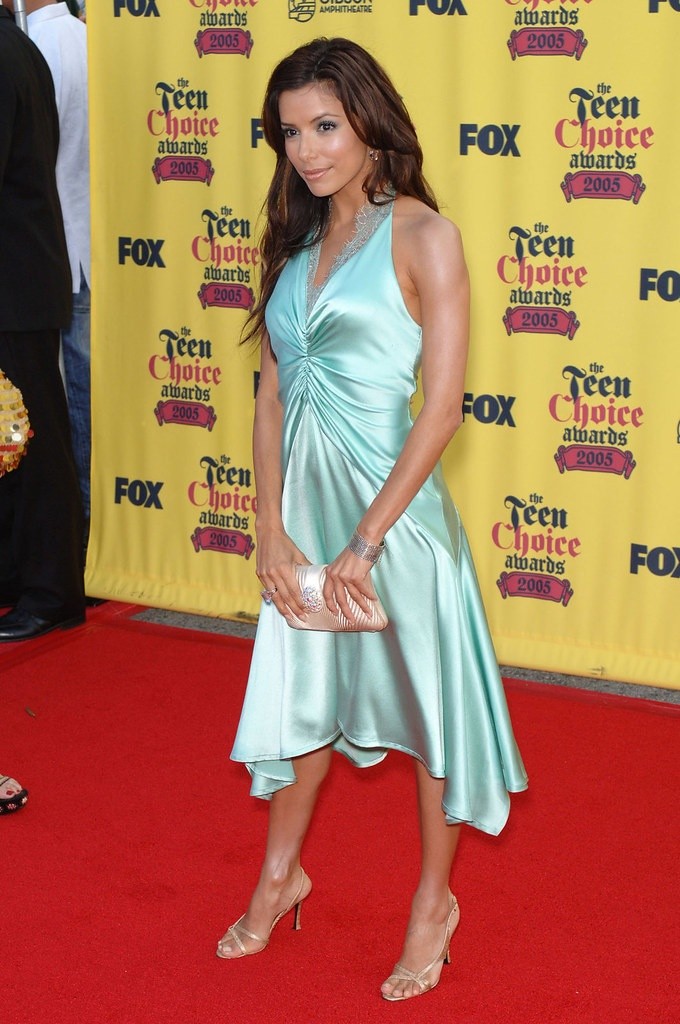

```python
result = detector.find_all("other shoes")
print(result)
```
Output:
[0,769,32,815]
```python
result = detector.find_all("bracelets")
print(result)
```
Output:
[348,530,385,562]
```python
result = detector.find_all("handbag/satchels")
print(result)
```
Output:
[272,562,388,636]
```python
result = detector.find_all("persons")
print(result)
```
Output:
[217,36,527,1001]
[0,6,85,643]
[3,0,90,523]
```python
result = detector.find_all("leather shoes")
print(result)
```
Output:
[0,606,86,642]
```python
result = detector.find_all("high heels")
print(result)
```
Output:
[380,893,461,1003]
[216,866,312,959]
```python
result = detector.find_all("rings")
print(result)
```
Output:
[260,588,278,606]
